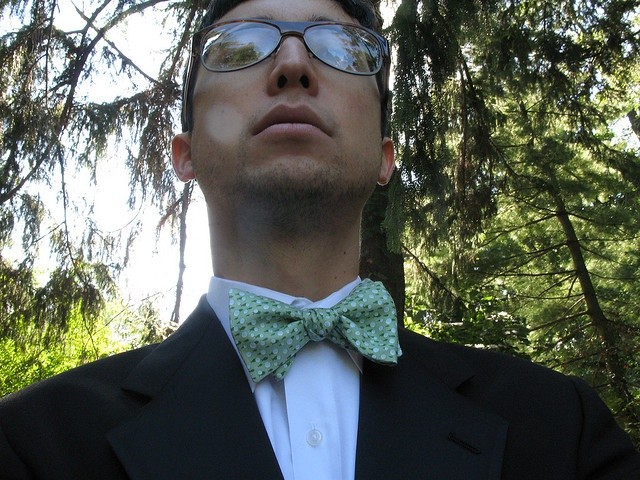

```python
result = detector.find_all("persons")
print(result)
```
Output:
[0,2,638,480]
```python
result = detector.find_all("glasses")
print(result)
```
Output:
[182,18,391,129]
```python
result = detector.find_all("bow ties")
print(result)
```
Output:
[228,278,399,383]
